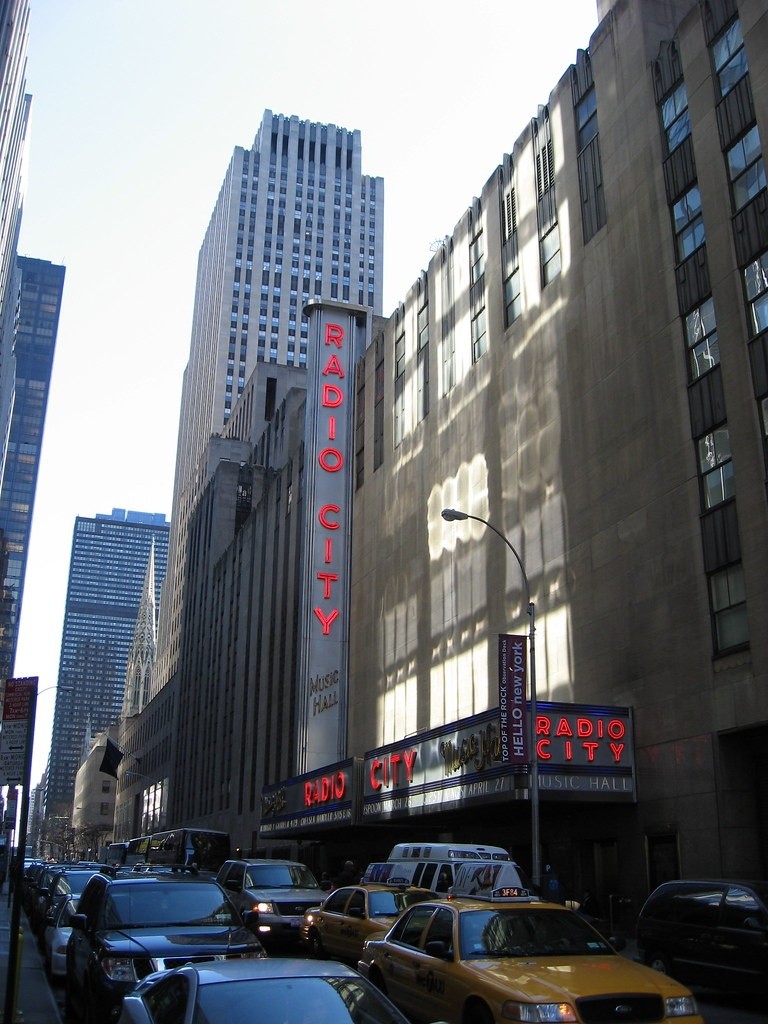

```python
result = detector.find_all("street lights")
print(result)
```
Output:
[441,509,542,894]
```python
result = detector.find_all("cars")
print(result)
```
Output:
[633,879,768,996]
[299,863,443,961]
[12,855,114,983]
[215,858,333,944]
[116,957,411,1024]
[358,861,705,1024]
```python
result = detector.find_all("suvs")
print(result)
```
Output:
[65,864,269,1024]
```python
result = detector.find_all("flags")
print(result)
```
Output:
[99,739,125,781]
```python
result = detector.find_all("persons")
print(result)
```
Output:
[321,871,336,895]
[337,859,356,886]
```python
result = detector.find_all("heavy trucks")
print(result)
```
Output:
[98,843,130,865]
[125,829,231,874]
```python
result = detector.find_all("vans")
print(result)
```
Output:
[387,843,515,900]
